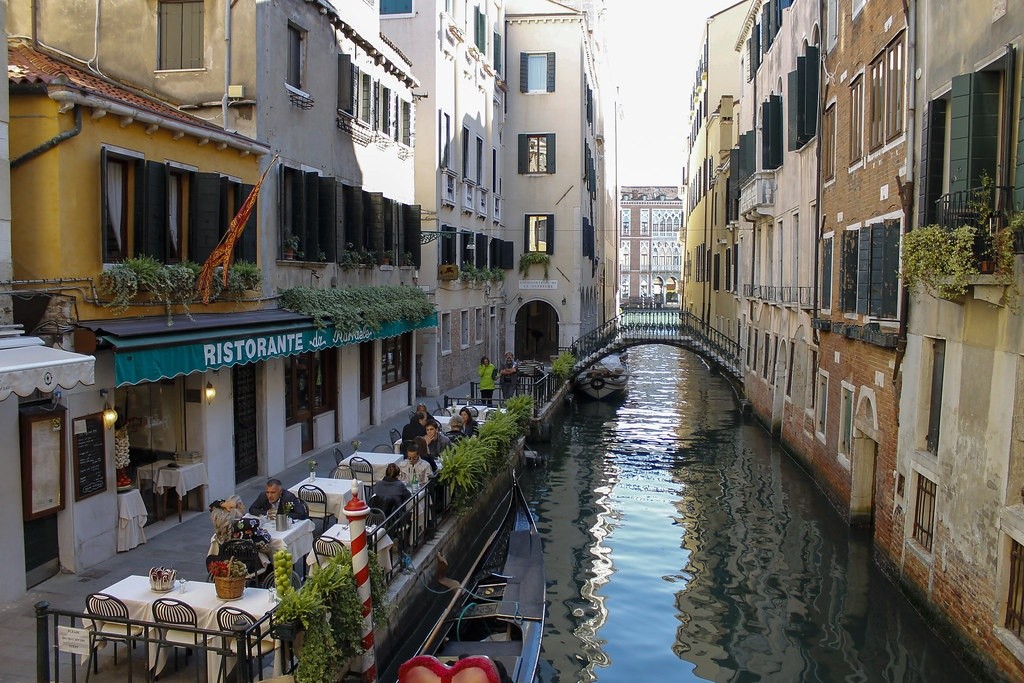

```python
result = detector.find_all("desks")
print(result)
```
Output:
[432,416,479,432]
[207,513,316,582]
[483,408,507,420]
[445,404,487,420]
[339,452,404,482]
[405,485,433,545]
[305,521,394,578]
[117,489,148,551]
[137,459,209,523]
[81,575,280,683]
[287,475,367,525]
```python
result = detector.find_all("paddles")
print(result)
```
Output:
[418,529,498,656]
[617,374,639,377]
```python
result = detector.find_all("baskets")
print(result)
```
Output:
[213,556,244,600]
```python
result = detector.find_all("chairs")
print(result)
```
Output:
[151,598,215,683]
[217,607,274,683]
[85,592,143,683]
[205,401,505,589]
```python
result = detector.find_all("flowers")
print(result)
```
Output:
[351,440,362,451]
[283,501,295,514]
[208,559,248,577]
[308,460,317,472]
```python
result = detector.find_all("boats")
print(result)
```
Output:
[574,348,630,402]
[394,468,547,683]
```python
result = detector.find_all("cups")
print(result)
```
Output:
[276,513,287,531]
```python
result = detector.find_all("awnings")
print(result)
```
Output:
[76,298,438,388]
[0,345,96,403]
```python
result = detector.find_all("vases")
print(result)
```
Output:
[355,450,358,457]
[310,471,315,481]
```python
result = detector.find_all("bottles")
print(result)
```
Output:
[411,472,418,493]
[179,577,186,594]
[268,588,274,603]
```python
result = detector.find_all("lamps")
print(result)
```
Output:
[420,230,476,249]
[100,389,118,430]
[517,297,522,303]
[561,298,566,305]
[483,283,491,295]
[205,381,216,406]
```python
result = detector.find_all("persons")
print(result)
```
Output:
[421,421,453,459]
[478,355,496,404]
[445,413,468,443]
[370,463,411,554]
[498,350,519,400]
[459,407,479,438]
[395,445,434,488]
[206,496,272,585]
[417,402,435,422]
[248,479,307,520]
[402,436,437,472]
[398,411,428,459]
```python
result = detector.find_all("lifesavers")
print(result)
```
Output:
[591,376,605,390]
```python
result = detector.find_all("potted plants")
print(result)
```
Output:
[519,251,550,281]
[383,250,395,265]
[282,233,304,260]
[97,253,265,327]
[269,544,390,683]
[967,168,1009,274]
